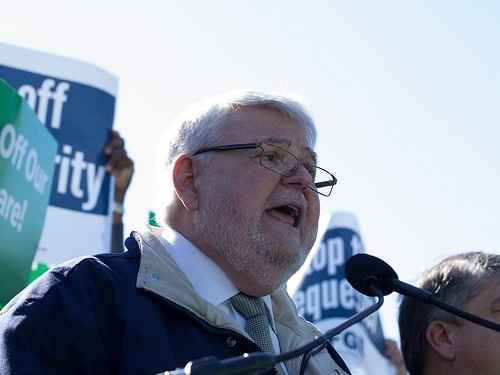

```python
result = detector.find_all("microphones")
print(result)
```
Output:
[185,253,500,375]
